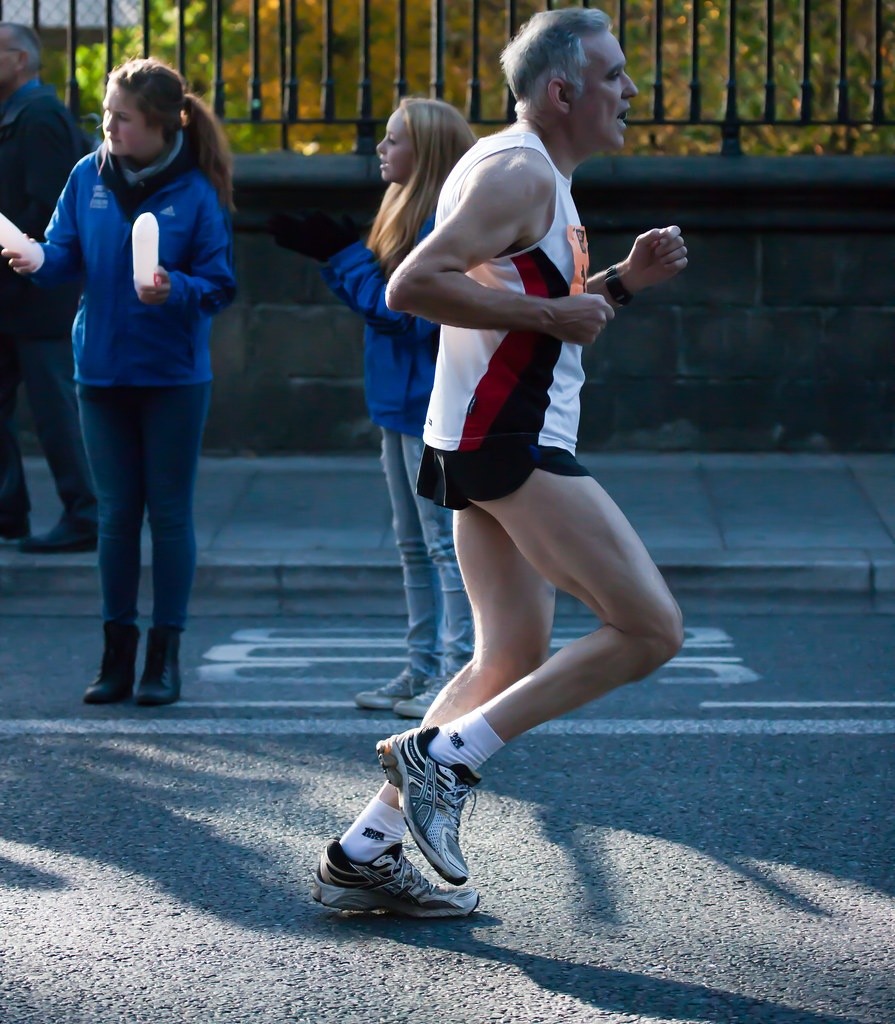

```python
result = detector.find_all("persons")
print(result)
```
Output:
[264,95,474,718]
[310,7,688,918]
[0,21,99,552]
[1,58,238,706]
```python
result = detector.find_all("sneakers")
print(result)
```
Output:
[375,726,483,885]
[394,678,450,718]
[309,836,481,918]
[355,665,431,709]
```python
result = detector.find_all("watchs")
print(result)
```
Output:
[604,264,634,306]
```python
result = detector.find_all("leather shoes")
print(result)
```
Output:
[83,621,140,705]
[134,627,181,705]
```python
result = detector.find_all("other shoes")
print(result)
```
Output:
[0,515,30,539]
[21,524,98,552]
